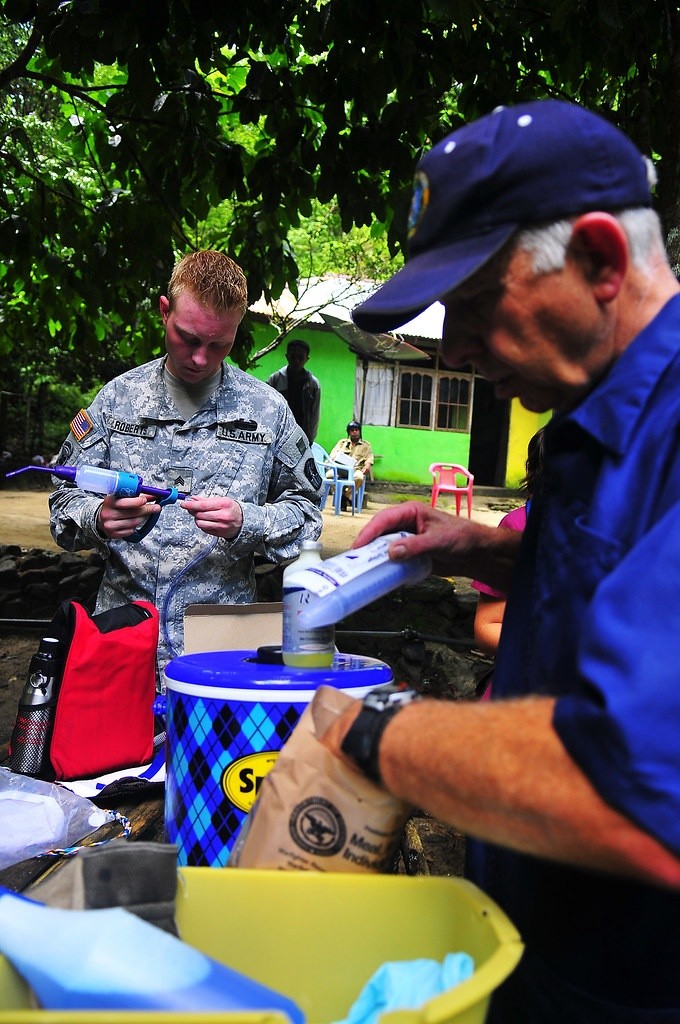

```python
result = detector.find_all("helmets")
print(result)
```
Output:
[347,421,361,432]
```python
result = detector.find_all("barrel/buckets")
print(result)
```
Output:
[165,650,395,867]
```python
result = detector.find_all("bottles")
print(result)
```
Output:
[10,638,59,775]
[282,540,335,667]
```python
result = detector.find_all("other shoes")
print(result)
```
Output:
[341,495,347,511]
[346,497,352,506]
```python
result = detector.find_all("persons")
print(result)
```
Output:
[48,250,323,736]
[318,100,680,1024]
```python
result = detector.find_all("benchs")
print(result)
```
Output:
[369,455,384,482]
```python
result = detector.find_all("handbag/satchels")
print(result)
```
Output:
[10,596,159,782]
[334,451,357,479]
[0,766,132,872]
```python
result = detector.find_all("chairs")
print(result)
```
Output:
[311,441,366,516]
[428,463,474,519]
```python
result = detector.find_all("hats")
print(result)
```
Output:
[352,101,657,334]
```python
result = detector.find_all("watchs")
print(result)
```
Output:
[341,685,427,790]
